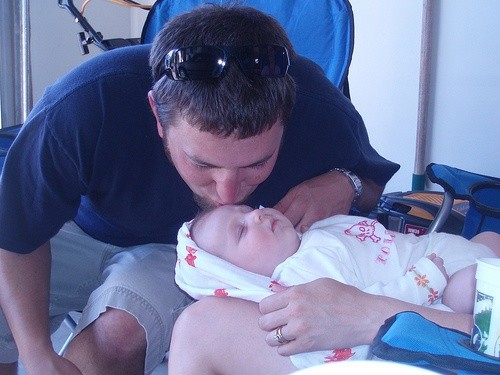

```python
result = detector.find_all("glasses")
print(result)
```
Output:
[157,42,291,82]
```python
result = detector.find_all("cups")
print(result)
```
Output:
[469,257,500,358]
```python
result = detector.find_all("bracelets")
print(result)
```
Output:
[330,167,362,203]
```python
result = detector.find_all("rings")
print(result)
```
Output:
[273,326,289,345]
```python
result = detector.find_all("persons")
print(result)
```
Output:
[174,204,500,314]
[166,277,474,375]
[0,6,401,375]
